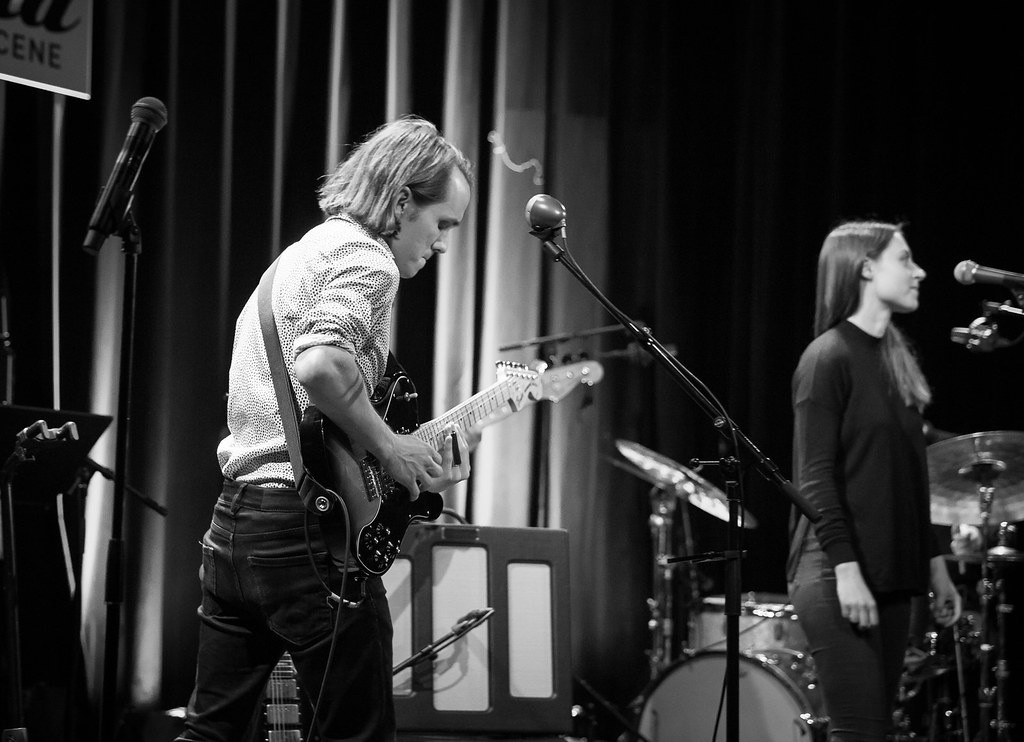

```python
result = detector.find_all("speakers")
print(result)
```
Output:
[380,524,574,742]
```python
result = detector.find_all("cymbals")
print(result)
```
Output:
[615,437,761,530]
[926,430,1024,526]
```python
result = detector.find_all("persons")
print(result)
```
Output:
[171,118,471,742]
[785,221,963,742]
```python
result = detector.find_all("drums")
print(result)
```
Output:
[634,645,829,742]
[931,552,1023,618]
[693,588,817,679]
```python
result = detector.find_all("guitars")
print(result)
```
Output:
[298,352,606,579]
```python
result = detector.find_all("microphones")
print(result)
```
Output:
[954,260,1024,289]
[83,96,168,257]
[612,343,678,366]
[950,327,1010,348]
[524,193,567,240]
[457,608,494,625]
